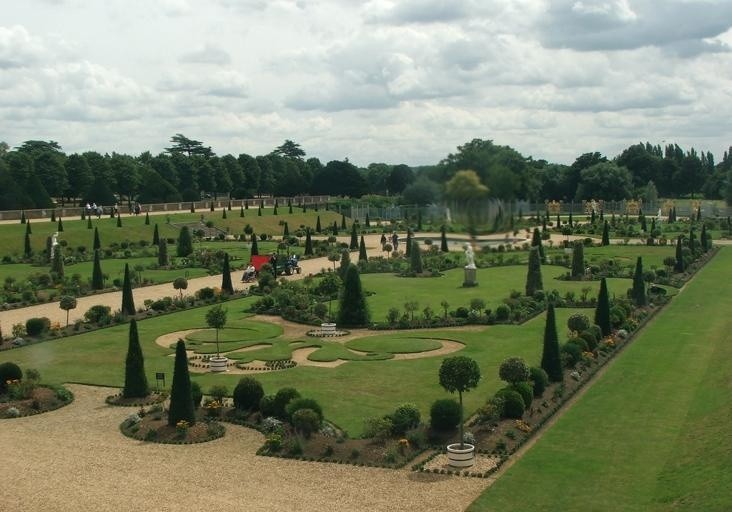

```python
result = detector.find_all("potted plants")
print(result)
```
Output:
[441,353,481,470]
[205,302,228,372]
[561,225,573,257]
[320,271,341,337]
[541,229,552,265]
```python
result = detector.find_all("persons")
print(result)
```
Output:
[463,246,477,270]
[380,233,386,248]
[245,264,255,281]
[270,252,277,268]
[241,264,250,281]
[84,202,142,218]
[392,231,399,250]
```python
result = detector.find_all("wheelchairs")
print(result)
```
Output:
[246,272,256,283]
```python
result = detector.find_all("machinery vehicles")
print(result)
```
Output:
[251,242,301,276]
